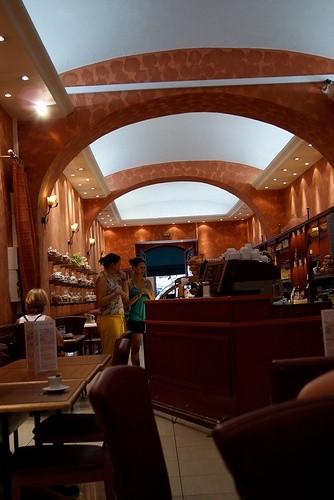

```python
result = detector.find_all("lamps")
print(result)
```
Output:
[41,195,58,224]
[67,223,79,245]
[87,238,95,256]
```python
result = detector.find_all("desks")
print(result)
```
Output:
[0,366,101,382]
[0,381,89,451]
[61,334,85,351]
[0,353,112,367]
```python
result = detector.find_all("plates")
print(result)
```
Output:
[64,336,73,339]
[42,385,69,392]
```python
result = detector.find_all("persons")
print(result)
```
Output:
[15,288,64,346]
[127,258,154,366]
[96,253,128,366]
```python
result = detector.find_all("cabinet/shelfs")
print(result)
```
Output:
[47,249,98,305]
[253,206,334,285]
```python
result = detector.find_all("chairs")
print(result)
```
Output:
[0,315,171,500]
[207,356,334,500]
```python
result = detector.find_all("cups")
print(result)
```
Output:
[87,314,95,323]
[47,376,62,389]
[60,325,65,337]
[224,244,271,263]
